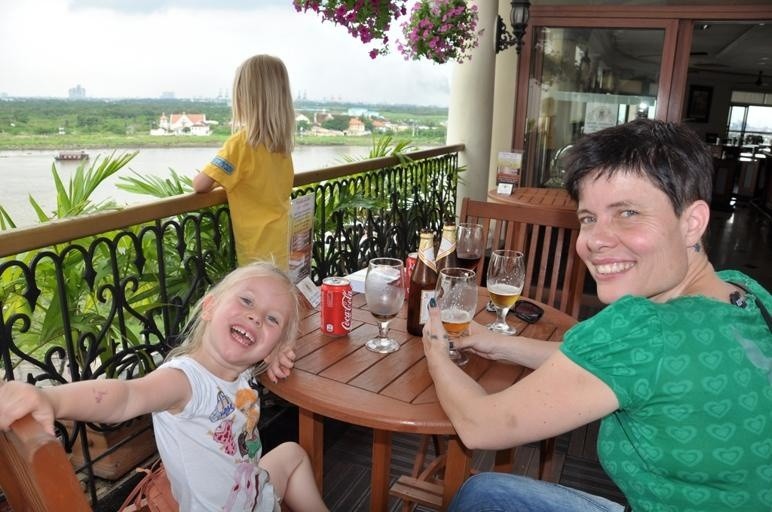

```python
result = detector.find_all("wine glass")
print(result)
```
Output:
[434,267,478,368]
[364,256,407,355]
[457,223,484,271]
[486,249,526,336]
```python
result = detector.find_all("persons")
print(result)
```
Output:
[0,256,330,512]
[192,53,302,292]
[419,116,772,512]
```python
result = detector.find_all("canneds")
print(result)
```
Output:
[320,278,352,337]
[405,252,418,303]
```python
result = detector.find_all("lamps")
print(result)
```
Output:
[496,0,531,55]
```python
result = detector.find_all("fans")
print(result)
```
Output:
[736,70,769,88]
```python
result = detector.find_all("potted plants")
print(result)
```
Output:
[0,150,189,480]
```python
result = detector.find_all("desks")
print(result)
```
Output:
[488,188,602,305]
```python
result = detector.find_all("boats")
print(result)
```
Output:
[54,151,89,161]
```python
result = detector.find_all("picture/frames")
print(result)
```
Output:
[687,84,714,123]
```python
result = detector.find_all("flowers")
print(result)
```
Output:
[394,0,484,64]
[292,0,406,65]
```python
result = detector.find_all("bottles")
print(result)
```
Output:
[407,216,457,336]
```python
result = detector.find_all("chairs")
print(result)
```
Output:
[433,198,585,482]
[0,378,146,512]
